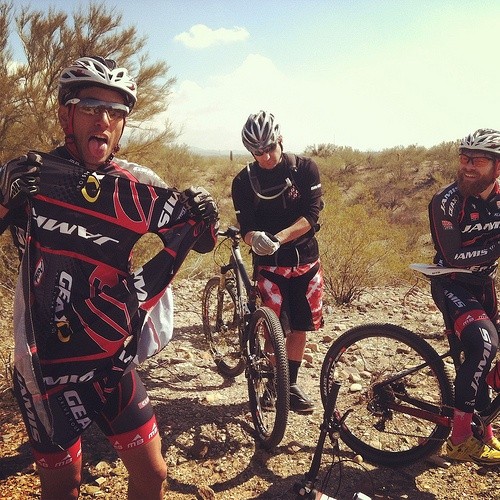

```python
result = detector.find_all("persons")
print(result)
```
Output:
[0,56,220,500]
[232,112,327,411]
[428,129,500,465]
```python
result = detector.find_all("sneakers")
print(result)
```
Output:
[446,436,500,465]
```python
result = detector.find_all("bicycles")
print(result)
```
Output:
[292,379,368,495]
[199,224,291,450]
[317,261,500,468]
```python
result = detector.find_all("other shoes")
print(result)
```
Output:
[262,387,277,411]
[289,385,317,412]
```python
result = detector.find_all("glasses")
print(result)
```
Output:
[63,97,130,119]
[458,153,495,169]
[254,139,278,156]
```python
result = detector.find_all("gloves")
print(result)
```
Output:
[250,230,281,257]
[178,186,220,224]
[0,152,43,211]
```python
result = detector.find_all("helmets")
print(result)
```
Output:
[241,110,279,152]
[57,55,138,113]
[458,128,500,154]
